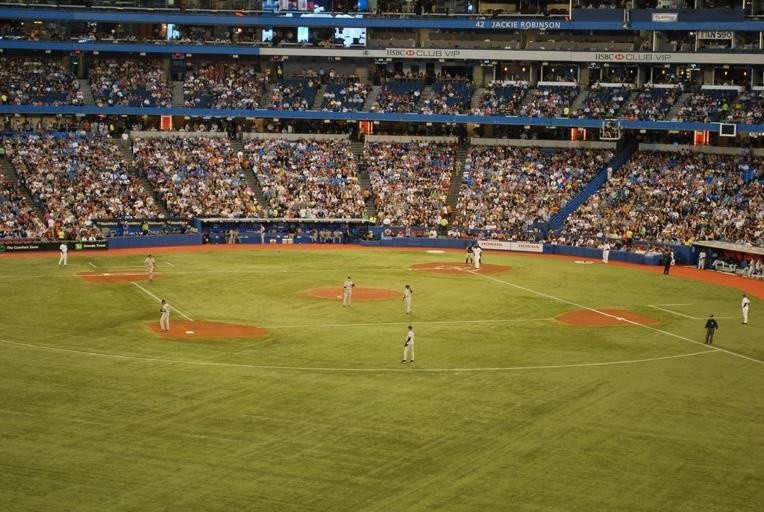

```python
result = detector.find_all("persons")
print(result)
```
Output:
[402,284,412,314]
[343,276,354,307]
[704,314,719,344]
[740,294,750,324]
[1,2,762,282]
[159,299,170,333]
[401,326,414,364]
